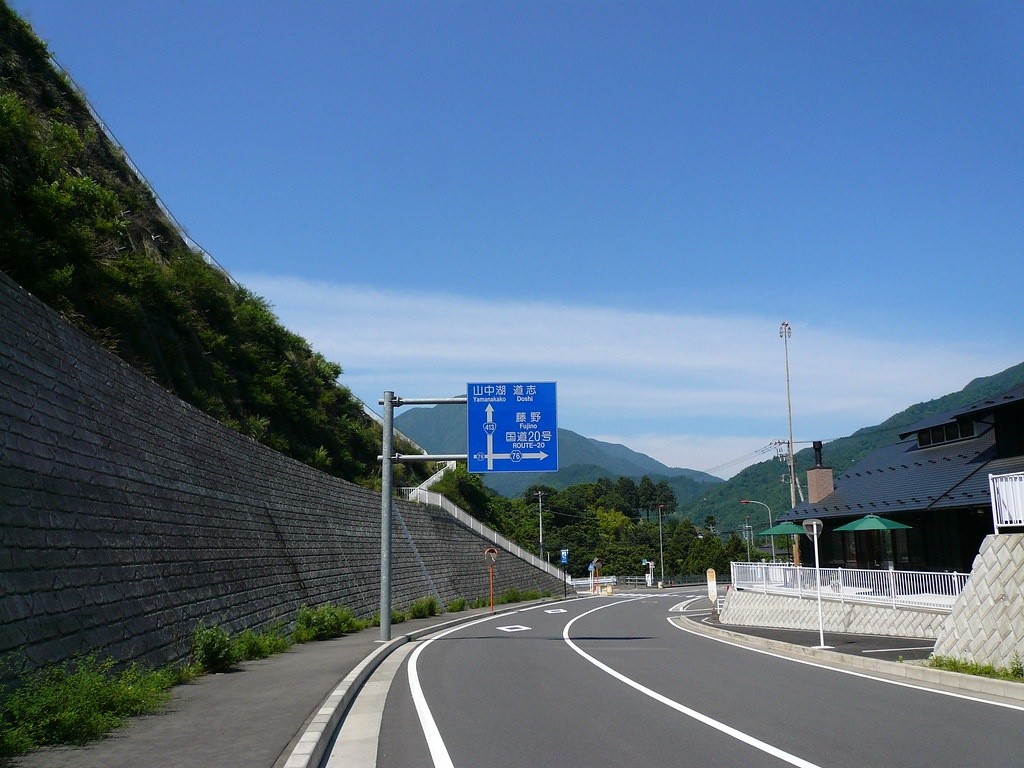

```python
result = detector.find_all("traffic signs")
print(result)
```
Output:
[466,381,558,474]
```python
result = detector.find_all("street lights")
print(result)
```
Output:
[780,322,800,566]
[658,503,664,585]
[745,515,751,562]
[741,500,775,562]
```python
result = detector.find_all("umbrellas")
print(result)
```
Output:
[833,513,912,562]
[757,522,807,560]
